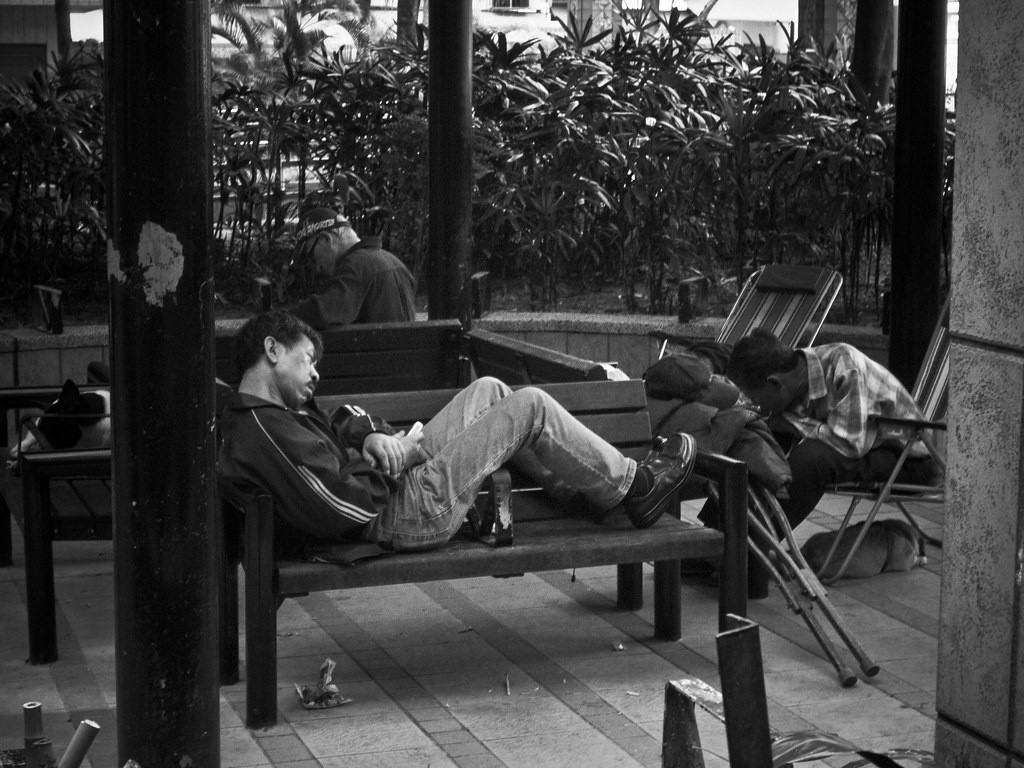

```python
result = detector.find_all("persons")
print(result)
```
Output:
[694,327,943,599]
[213,309,699,568]
[280,207,417,330]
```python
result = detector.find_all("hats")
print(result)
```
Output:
[642,353,740,409]
[289,208,352,271]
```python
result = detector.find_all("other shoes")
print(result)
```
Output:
[680,558,720,586]
[748,571,768,600]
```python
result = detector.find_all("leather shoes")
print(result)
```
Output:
[623,432,697,529]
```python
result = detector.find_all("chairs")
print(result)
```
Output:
[814,289,952,587]
[648,263,843,361]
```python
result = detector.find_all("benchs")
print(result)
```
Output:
[463,324,644,612]
[215,379,748,729]
[0,382,113,667]
[82,318,471,396]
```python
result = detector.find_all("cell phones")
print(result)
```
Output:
[407,421,424,436]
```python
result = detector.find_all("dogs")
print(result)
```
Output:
[786,519,928,583]
[6,379,111,471]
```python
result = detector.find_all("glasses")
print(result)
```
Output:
[304,236,320,264]
